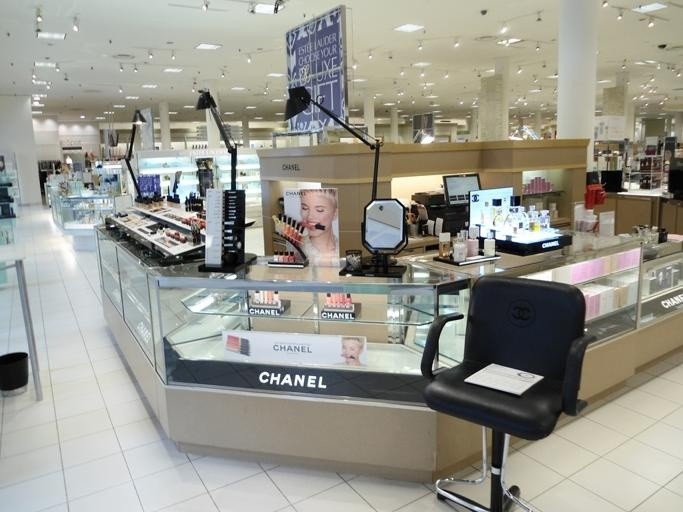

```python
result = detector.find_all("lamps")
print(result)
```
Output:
[125,87,381,201]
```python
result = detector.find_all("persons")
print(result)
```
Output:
[283,190,338,256]
[339,336,365,366]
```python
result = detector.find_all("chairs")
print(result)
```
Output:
[420,275,598,512]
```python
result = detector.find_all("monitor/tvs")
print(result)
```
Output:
[442,172,481,220]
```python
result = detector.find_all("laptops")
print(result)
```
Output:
[601,170,628,192]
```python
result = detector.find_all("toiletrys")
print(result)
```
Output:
[438,206,552,267]
[325,291,352,308]
[252,290,279,304]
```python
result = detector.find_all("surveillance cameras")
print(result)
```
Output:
[658,43,667,50]
[480,8,488,15]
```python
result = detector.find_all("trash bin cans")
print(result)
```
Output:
[0,352,28,397]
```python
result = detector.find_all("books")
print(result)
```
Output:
[465,362,544,397]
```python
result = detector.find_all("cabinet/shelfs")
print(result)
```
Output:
[46,139,683,484]
[0,156,20,245]
